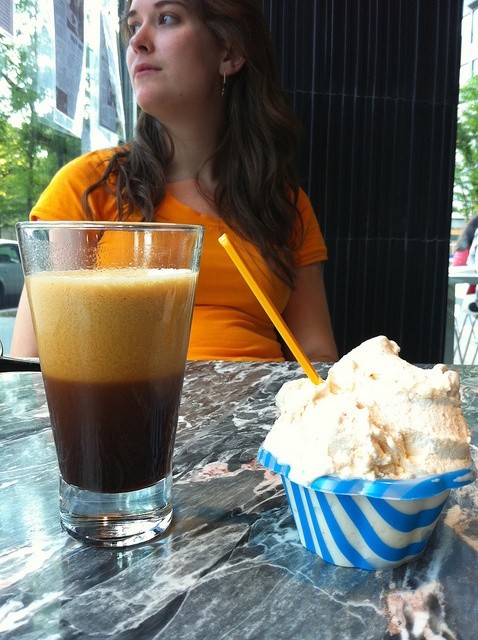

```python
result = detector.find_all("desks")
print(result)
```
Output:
[0,362,478,640]
[442,265,478,365]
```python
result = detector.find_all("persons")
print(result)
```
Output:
[455,213,478,311]
[10,0,339,367]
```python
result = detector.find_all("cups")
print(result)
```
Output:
[14,221,205,547]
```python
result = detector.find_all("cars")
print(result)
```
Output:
[0,238,25,306]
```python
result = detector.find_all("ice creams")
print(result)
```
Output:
[262,336,472,484]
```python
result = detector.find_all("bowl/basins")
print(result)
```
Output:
[256,439,478,569]
